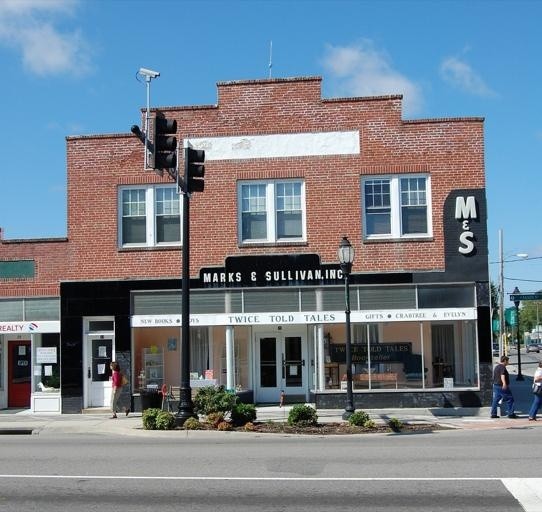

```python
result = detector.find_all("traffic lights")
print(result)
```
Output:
[150,108,178,169]
[184,140,205,193]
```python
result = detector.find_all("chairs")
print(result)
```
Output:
[402,354,427,381]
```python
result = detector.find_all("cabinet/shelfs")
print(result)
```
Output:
[142,348,165,387]
[325,362,339,388]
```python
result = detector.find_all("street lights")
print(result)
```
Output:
[335,232,355,420]
[497,229,528,357]
[510,287,524,382]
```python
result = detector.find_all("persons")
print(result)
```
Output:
[110,362,131,418]
[528,361,542,421]
[491,356,519,419]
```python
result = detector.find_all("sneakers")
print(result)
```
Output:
[491,413,536,421]
[109,408,130,419]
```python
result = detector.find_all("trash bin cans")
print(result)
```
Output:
[140,389,162,411]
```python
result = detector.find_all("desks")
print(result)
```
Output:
[352,373,398,389]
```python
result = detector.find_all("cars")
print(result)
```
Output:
[493,343,499,355]
[526,343,542,354]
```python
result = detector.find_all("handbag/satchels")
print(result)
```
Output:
[121,375,128,385]
[531,384,542,395]
